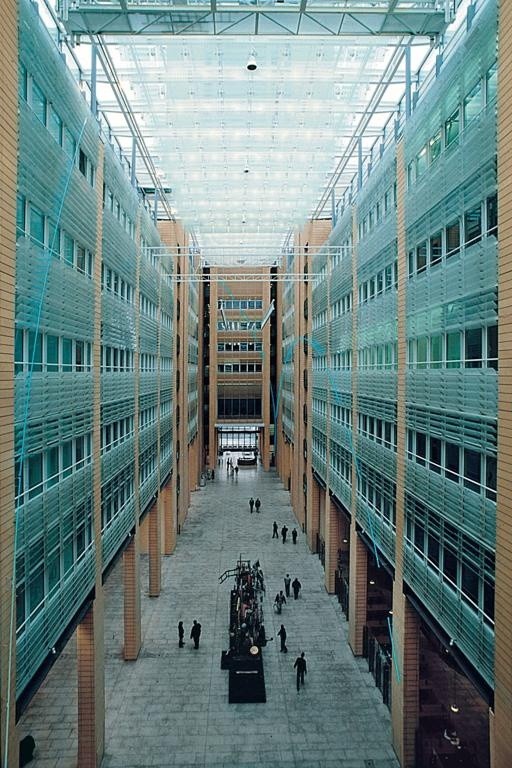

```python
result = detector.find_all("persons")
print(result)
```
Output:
[277,624,288,653]
[220,449,244,478]
[190,619,202,649]
[283,573,291,597]
[255,497,260,512]
[291,527,298,544]
[292,652,307,692]
[273,590,287,613]
[177,621,187,647]
[291,577,302,599]
[271,521,279,539]
[280,523,289,544]
[248,497,253,512]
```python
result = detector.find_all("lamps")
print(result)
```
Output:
[246,36,258,72]
